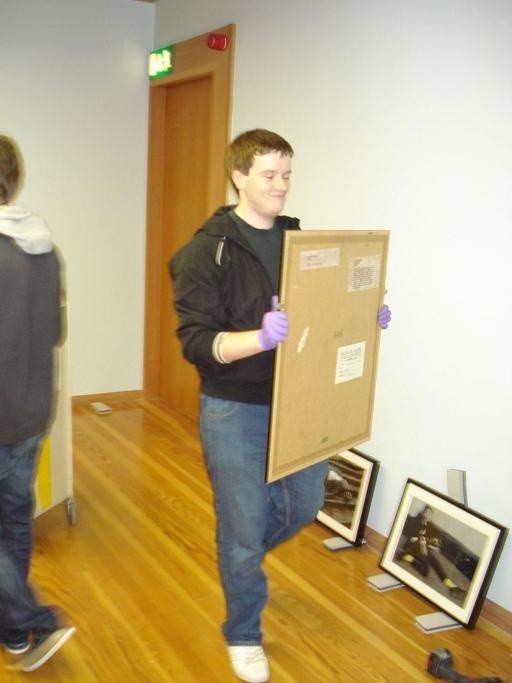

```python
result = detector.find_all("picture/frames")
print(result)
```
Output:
[265,229,391,485]
[378,477,509,630]
[313,450,381,546]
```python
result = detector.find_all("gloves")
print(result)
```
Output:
[377,304,392,330]
[256,294,290,353]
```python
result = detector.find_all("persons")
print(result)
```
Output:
[0,133,75,671]
[397,507,468,601]
[167,130,390,683]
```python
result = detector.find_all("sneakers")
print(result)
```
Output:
[449,586,469,601]
[396,549,409,562]
[19,625,77,673]
[226,615,272,682]
[1,607,32,656]
